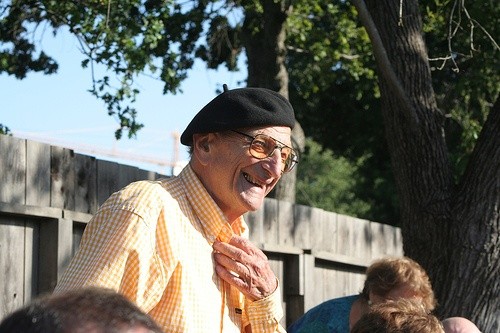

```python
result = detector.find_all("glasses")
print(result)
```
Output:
[227,127,298,173]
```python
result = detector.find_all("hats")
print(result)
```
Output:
[181,84,294,146]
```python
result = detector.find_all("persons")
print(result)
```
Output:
[286,256,483,333]
[50,87,299,332]
